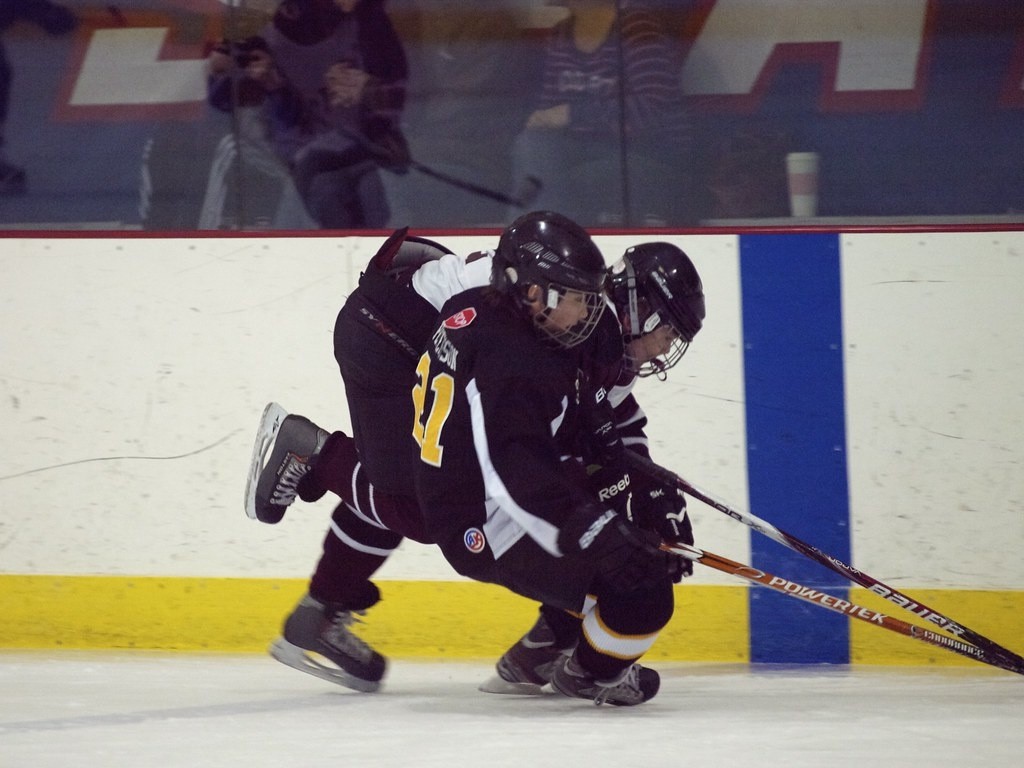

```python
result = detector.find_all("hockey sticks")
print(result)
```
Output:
[268,83,551,208]
[625,442,1024,682]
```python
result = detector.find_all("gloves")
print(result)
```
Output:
[593,466,651,531]
[641,476,695,582]
[367,116,411,174]
[564,503,657,589]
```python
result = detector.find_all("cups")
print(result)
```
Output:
[786,152,820,217]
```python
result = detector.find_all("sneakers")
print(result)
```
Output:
[269,591,386,693]
[245,402,329,524]
[548,647,660,706]
[479,615,574,696]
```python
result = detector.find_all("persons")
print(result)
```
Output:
[128,0,727,231]
[204,0,416,229]
[241,239,705,691]
[401,209,675,709]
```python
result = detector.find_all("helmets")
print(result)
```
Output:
[604,242,706,378]
[489,210,608,354]
